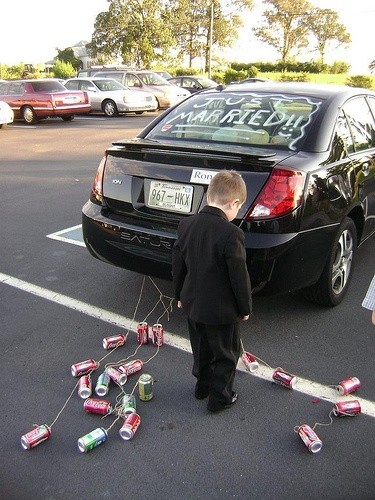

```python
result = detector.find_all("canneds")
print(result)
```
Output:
[119,412,141,440]
[103,334,125,349]
[20,424,50,450]
[71,359,96,377]
[152,324,163,346]
[119,359,143,375]
[138,374,153,401]
[77,426,108,452]
[336,376,361,395]
[78,366,136,418]
[272,370,297,388]
[333,400,361,416]
[137,322,149,344]
[297,424,322,453]
[242,352,259,373]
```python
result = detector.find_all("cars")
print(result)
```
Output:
[77,68,190,109]
[0,102,15,127]
[0,79,90,125]
[61,77,159,118]
[80,81,375,308]
[166,76,217,94]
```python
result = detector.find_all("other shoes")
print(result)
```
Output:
[220,392,239,407]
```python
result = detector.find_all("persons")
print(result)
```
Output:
[361,275,375,325]
[171,169,255,416]
